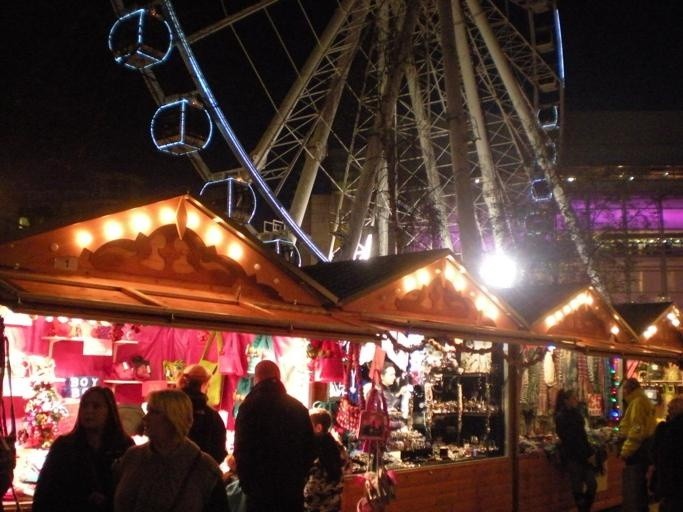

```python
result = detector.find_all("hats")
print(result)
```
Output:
[132,356,150,366]
[249,362,280,379]
[185,367,211,381]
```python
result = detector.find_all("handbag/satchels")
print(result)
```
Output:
[337,398,397,512]
[0,437,16,496]
[198,345,276,419]
[312,357,343,383]
[573,444,606,470]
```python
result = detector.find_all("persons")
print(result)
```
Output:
[26,386,135,512]
[612,376,657,511]
[303,407,345,512]
[552,388,600,511]
[233,360,314,511]
[625,396,683,512]
[89,388,230,512]
[175,364,229,467]
